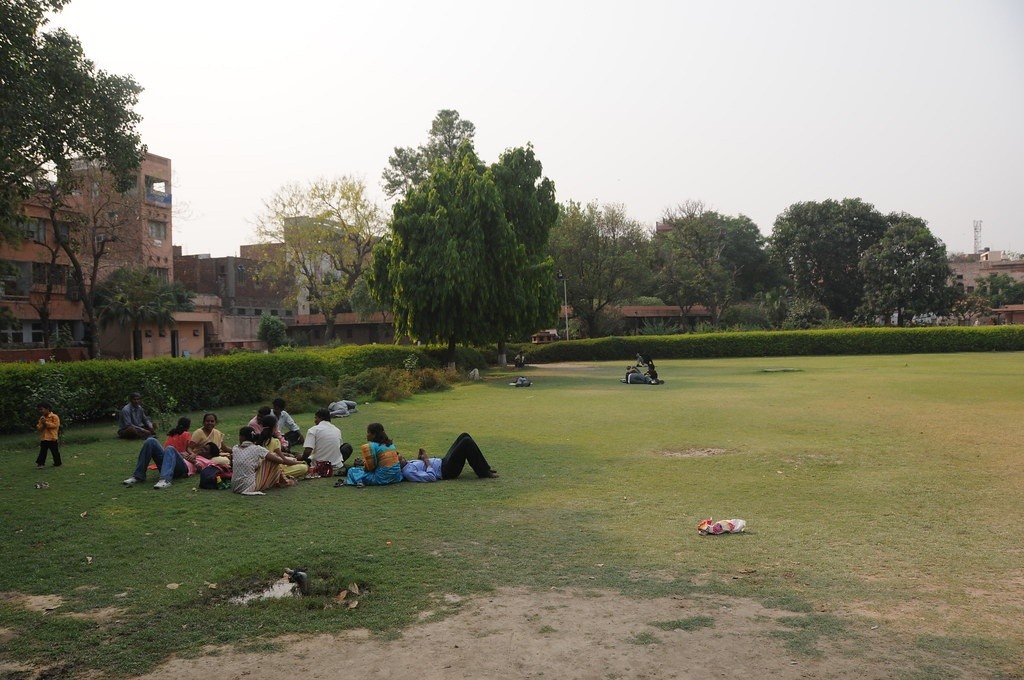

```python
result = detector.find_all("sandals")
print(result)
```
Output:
[333,478,345,487]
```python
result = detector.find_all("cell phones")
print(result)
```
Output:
[419,449,422,455]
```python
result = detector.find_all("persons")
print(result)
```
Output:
[624,350,664,385]
[514,350,525,369]
[34,402,62,470]
[117,391,500,494]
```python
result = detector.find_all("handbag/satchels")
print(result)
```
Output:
[199,464,222,490]
[313,460,333,477]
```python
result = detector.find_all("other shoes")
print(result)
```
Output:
[490,469,497,473]
[36,464,45,468]
[489,472,499,478]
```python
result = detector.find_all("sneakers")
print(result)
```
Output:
[123,476,139,484]
[153,480,171,488]
